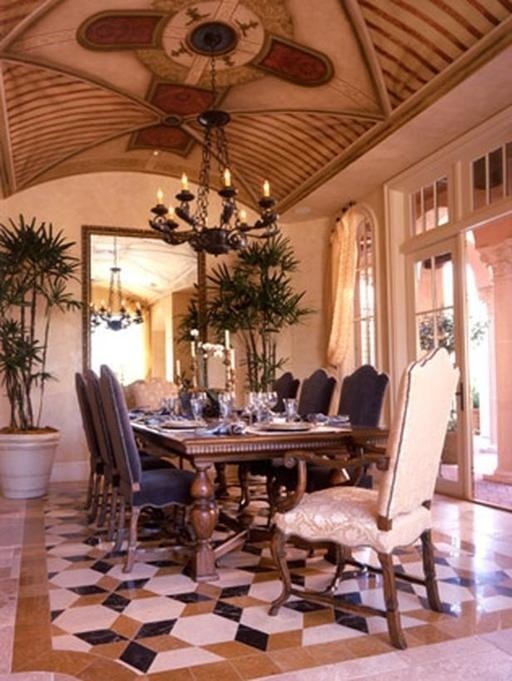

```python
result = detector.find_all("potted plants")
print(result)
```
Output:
[0,213,89,500]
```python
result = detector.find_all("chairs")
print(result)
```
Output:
[270,364,388,503]
[74,346,178,539]
[272,371,299,412]
[270,346,459,650]
[100,365,192,573]
[295,369,337,421]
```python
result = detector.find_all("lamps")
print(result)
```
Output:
[147,32,283,253]
[90,237,144,331]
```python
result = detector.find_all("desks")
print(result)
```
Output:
[127,408,389,583]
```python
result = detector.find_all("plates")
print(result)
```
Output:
[161,422,203,429]
[263,424,310,432]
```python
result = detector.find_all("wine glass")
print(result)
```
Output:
[188,391,298,424]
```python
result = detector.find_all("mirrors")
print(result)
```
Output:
[80,223,209,410]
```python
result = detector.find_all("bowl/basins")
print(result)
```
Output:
[309,415,329,426]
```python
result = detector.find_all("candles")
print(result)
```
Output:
[224,329,230,350]
[189,340,196,359]
